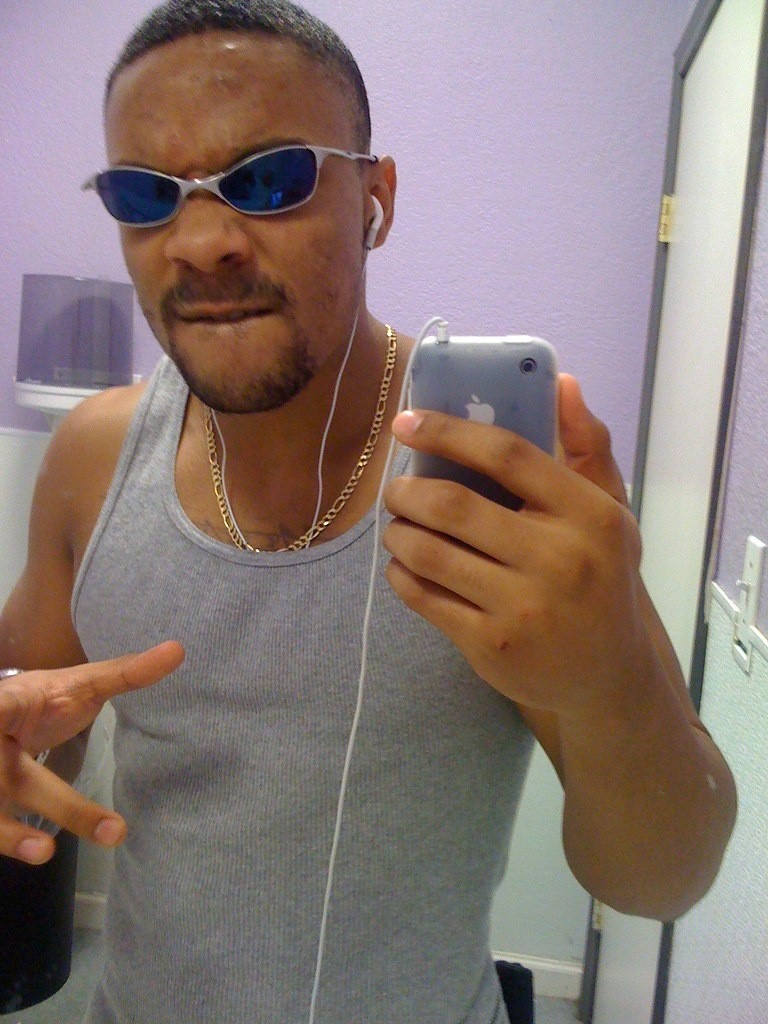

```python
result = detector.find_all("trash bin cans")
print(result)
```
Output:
[0,725,100,1016]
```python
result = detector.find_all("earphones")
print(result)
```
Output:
[366,195,384,252]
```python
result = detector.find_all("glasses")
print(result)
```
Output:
[81,144,379,228]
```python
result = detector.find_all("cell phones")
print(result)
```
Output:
[406,334,558,511]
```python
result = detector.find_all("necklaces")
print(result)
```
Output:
[204,325,396,555]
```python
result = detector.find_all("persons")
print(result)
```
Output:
[0,0,738,1024]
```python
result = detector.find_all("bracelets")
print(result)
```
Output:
[0,667,50,765]
